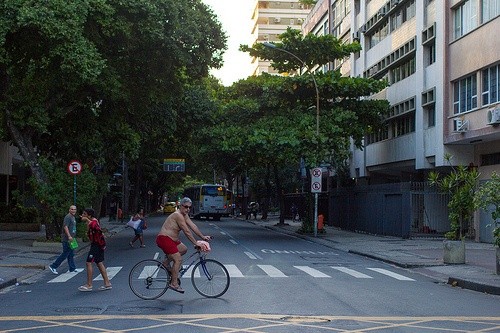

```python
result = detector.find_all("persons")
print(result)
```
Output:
[129,208,147,247]
[156,197,211,293]
[230,201,264,220]
[79,209,112,292]
[48,205,78,274]
[292,203,298,223]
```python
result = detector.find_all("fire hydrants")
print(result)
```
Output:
[317,215,325,234]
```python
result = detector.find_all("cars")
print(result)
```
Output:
[163,202,177,216]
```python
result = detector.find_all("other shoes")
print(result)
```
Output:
[129,241,135,248]
[140,244,146,247]
[49,266,58,275]
[74,269,78,272]
[169,284,184,294]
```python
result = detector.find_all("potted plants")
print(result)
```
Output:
[427,153,482,264]
[473,170,500,275]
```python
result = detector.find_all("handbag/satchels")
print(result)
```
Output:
[127,216,141,230]
[68,238,78,249]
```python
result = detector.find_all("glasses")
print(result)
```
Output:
[182,204,191,209]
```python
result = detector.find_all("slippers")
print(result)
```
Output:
[78,285,93,292]
[98,286,112,291]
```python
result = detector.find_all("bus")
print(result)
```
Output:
[183,184,228,221]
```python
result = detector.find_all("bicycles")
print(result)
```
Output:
[128,236,231,300]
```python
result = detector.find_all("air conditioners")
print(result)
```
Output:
[486,107,500,125]
[452,118,462,133]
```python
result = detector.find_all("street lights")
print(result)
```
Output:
[262,41,320,236]
[114,174,124,223]
